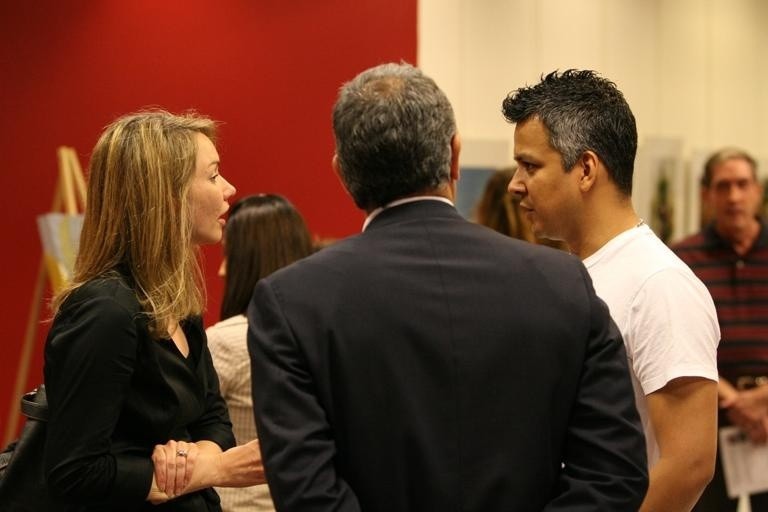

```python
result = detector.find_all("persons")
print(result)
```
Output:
[199,192,319,512]
[497,65,719,512]
[0,105,270,512]
[475,165,569,253]
[244,58,650,512]
[668,145,767,511]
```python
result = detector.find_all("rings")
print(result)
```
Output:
[175,448,187,458]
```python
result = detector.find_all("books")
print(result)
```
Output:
[715,427,767,499]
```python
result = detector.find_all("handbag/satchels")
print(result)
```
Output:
[0,381,58,511]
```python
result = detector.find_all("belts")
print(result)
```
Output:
[734,375,762,392]
